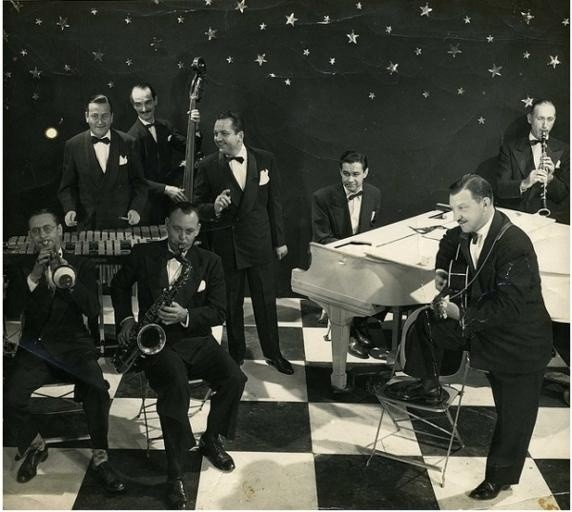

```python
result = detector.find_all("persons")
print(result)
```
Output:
[306,145,397,363]
[493,97,569,224]
[125,84,202,228]
[3,206,127,493]
[192,112,296,376]
[109,203,252,510]
[53,90,150,348]
[380,172,556,502]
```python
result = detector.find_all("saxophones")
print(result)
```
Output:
[109,251,191,372]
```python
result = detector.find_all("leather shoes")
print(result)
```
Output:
[199,435,235,471]
[348,327,374,358]
[384,380,443,404]
[167,478,188,510]
[88,458,126,492]
[17,444,48,483]
[470,479,510,499]
[266,353,294,375]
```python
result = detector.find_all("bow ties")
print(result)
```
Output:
[91,122,158,144]
[226,157,243,164]
[468,232,478,244]
[349,191,362,200]
[528,140,549,145]
[168,251,182,260]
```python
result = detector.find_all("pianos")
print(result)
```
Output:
[290,200,570,392]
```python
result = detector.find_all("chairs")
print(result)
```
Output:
[366,305,470,488]
[30,383,84,417]
[136,371,212,458]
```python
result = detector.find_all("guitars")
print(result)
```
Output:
[426,240,470,318]
